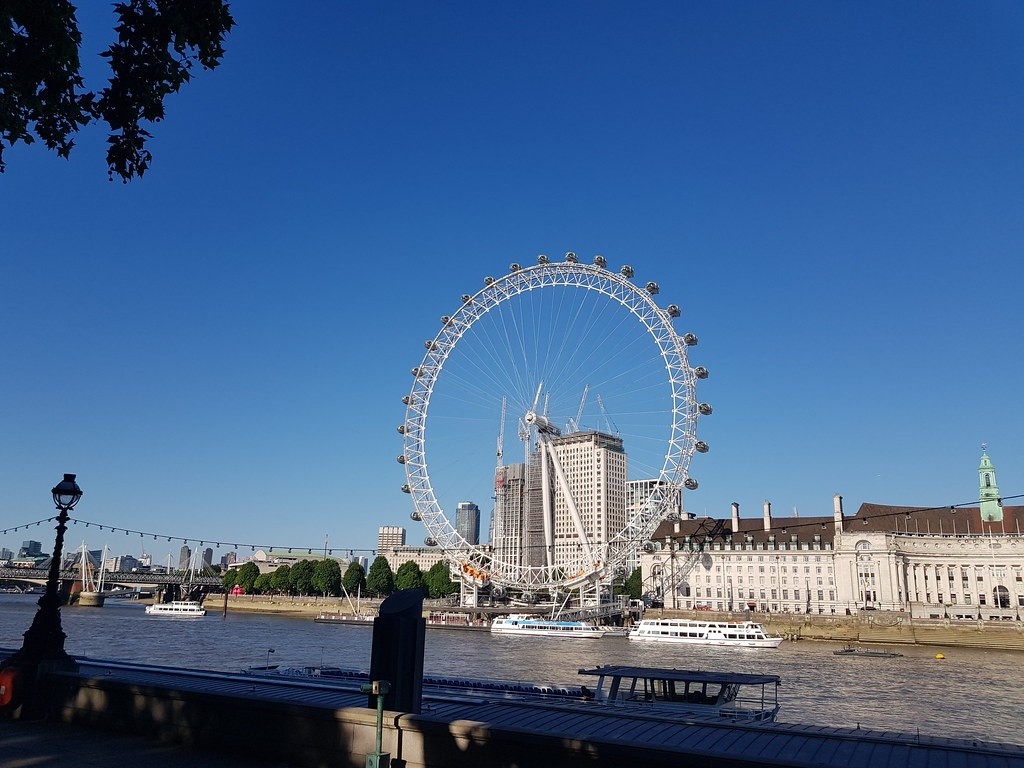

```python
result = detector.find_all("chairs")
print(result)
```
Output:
[542,688,546,694]
[547,688,553,694]
[538,689,541,693]
[525,687,529,692]
[587,689,594,697]
[554,689,557,695]
[529,686,533,692]
[568,691,571,696]
[572,690,577,697]
[581,686,589,697]
[561,689,568,695]
[320,670,499,691]
[576,690,582,697]
[533,687,538,693]
[500,684,504,690]
[510,686,513,691]
[513,685,518,691]
[557,689,561,696]
[505,685,509,690]
[518,686,523,691]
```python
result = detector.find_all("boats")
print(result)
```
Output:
[314,610,374,626]
[144,601,208,615]
[241,665,280,675]
[425,611,491,632]
[578,662,782,724]
[626,617,784,648]
[491,613,628,639]
[833,644,904,658]
[279,662,607,704]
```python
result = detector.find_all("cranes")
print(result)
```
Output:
[572,384,590,433]
[596,394,621,435]
[495,394,507,466]
